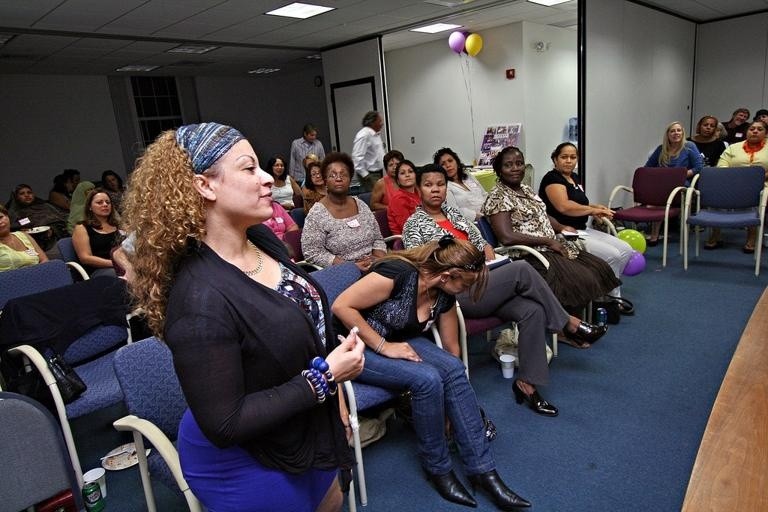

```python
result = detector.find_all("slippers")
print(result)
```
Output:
[704,239,719,250]
[742,243,756,253]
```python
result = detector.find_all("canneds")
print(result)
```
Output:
[596,307,607,327]
[82,481,105,512]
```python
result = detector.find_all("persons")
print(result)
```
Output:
[1,111,636,512]
[644,110,768,252]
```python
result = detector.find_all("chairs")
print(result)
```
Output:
[682,163,768,277]
[0,192,623,512]
[606,165,693,269]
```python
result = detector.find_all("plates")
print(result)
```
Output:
[99,442,150,470]
[27,225,50,235]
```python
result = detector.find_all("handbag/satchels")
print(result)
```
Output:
[9,339,88,408]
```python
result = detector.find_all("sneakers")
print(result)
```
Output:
[648,238,660,247]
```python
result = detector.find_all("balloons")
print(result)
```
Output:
[461,32,471,56]
[465,34,482,57]
[623,251,646,277]
[448,31,465,55]
[617,229,647,254]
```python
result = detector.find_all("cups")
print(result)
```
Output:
[499,354,515,379]
[83,466,106,499]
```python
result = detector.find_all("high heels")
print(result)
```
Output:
[420,464,477,508]
[564,320,609,348]
[469,469,532,509]
[511,378,559,418]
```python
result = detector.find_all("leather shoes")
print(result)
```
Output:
[594,294,635,326]
